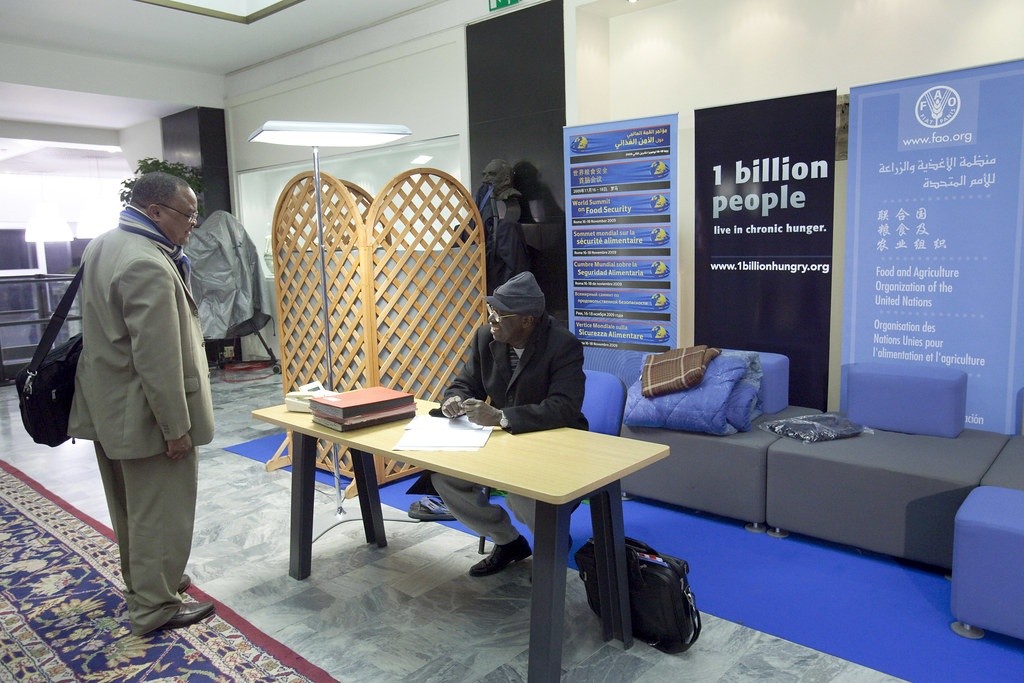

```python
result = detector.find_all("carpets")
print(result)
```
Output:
[0,458,340,683]
[220,432,1023,683]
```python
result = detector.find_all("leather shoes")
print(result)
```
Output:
[469,535,532,578]
[177,574,191,594]
[530,533,573,582]
[151,601,215,630]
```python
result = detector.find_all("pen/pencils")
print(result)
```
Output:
[451,397,475,421]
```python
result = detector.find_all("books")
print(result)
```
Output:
[309,386,417,432]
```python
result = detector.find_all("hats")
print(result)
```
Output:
[485,270,545,319]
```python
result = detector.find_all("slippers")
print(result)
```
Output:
[408,495,458,520]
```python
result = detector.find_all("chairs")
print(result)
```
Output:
[477,370,628,581]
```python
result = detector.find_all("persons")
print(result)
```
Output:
[67,172,215,636]
[482,158,521,224]
[429,271,588,576]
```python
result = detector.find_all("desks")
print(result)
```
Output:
[251,391,670,683]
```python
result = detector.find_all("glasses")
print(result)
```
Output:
[485,303,522,323]
[157,203,199,223]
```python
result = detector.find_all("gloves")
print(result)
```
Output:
[443,396,503,426]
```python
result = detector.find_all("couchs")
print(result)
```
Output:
[618,348,1024,641]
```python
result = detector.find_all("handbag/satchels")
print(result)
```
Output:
[573,534,703,654]
[14,330,83,447]
[455,219,532,289]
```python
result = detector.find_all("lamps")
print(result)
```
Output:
[247,120,421,544]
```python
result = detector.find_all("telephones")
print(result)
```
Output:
[284,381,341,413]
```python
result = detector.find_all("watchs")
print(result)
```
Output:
[499,410,509,428]
